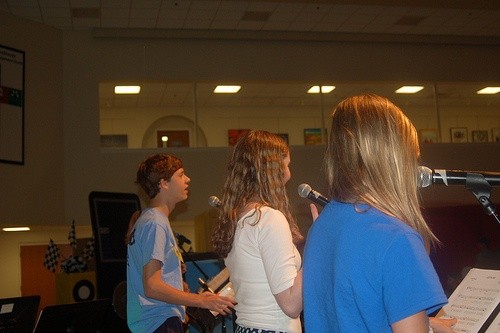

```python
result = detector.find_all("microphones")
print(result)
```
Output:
[173,231,191,244]
[297,183,330,207]
[416,166,500,188]
[208,195,222,208]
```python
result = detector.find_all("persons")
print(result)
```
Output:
[207,130,304,333]
[125,152,237,333]
[301,92,458,333]
